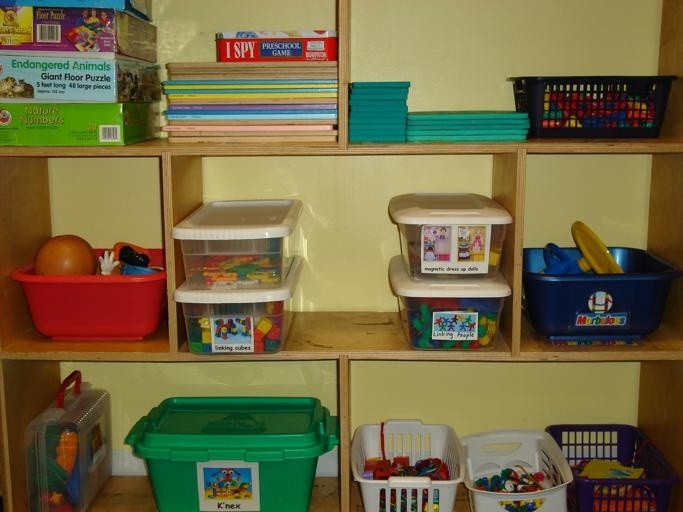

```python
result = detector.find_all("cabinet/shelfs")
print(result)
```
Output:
[0,1,683,512]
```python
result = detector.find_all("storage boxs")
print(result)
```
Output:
[506,74,679,138]
[387,254,512,349]
[388,192,511,278]
[123,394,341,512]
[173,198,301,292]
[543,424,683,512]
[523,245,678,345]
[350,418,467,512]
[175,254,300,353]
[12,248,167,342]
[462,429,574,512]
